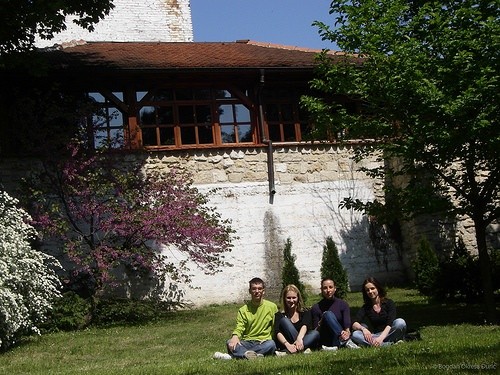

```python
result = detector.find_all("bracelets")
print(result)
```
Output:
[284,341,287,344]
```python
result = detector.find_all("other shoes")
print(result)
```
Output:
[275,350,288,358]
[303,348,313,354]
[347,340,362,350]
[394,340,404,346]
[213,352,231,360]
[244,350,265,360]
[320,345,338,352]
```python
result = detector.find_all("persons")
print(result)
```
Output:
[311,277,362,351]
[273,284,321,356]
[222,278,278,360]
[351,278,407,348]
